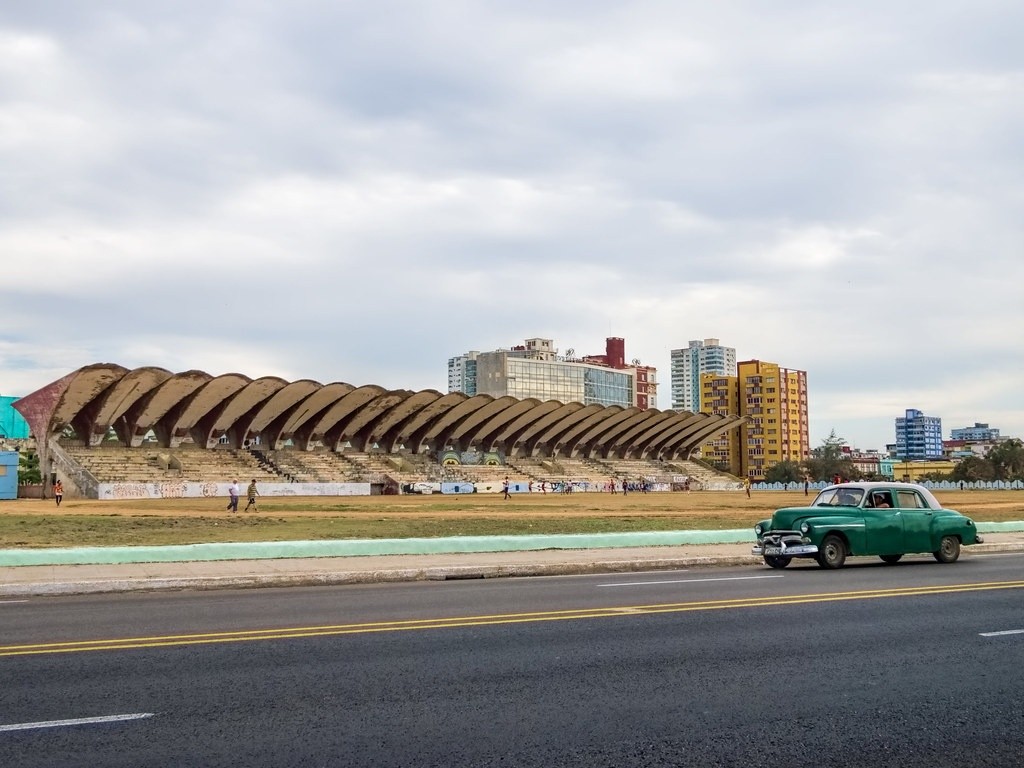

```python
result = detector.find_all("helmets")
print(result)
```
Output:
[875,494,885,499]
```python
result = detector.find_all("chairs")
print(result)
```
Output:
[837,494,857,504]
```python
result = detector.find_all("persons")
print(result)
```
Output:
[504,476,691,500]
[868,493,890,508]
[53,480,65,508]
[960,480,965,491]
[227,480,240,513]
[744,475,848,498]
[245,479,261,513]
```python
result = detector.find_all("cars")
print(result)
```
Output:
[752,481,984,570]
